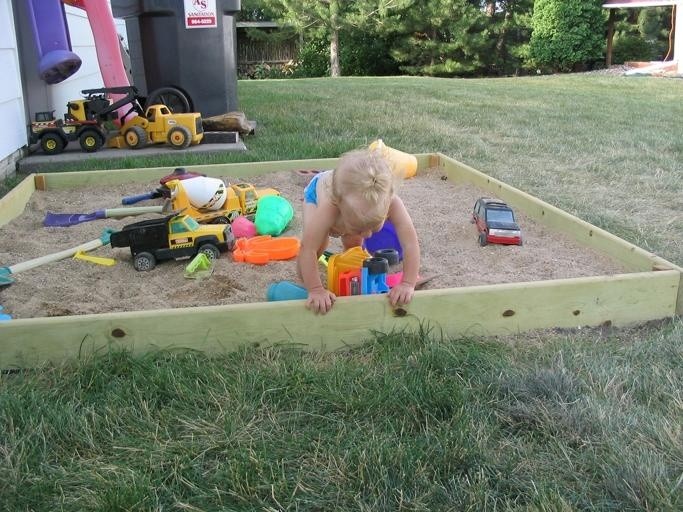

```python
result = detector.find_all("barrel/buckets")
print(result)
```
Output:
[255,196,293,235]
[367,138,417,180]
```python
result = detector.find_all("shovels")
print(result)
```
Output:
[0,231,117,295]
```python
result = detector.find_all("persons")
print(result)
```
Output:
[292,146,421,318]
